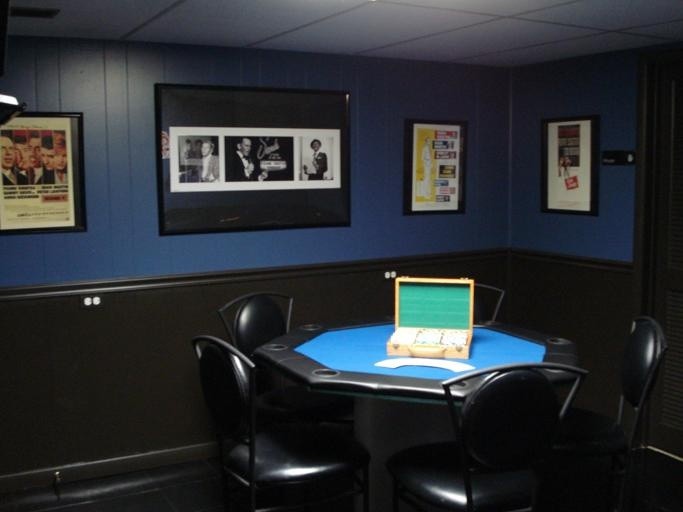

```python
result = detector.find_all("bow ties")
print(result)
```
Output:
[242,155,251,161]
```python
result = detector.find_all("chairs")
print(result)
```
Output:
[385,361,591,511]
[215,290,346,424]
[189,335,372,511]
[547,313,670,511]
[475,282,507,325]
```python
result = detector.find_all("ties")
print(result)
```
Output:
[312,153,317,161]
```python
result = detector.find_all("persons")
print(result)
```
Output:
[0,126,68,188]
[419,135,433,200]
[225,137,269,181]
[257,138,279,161]
[300,138,328,180]
[181,136,219,183]
[562,156,571,175]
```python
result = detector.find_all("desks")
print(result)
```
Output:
[255,316,585,510]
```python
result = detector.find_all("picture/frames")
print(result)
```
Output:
[540,115,602,220]
[0,113,87,234]
[401,118,466,215]
[151,81,354,236]
